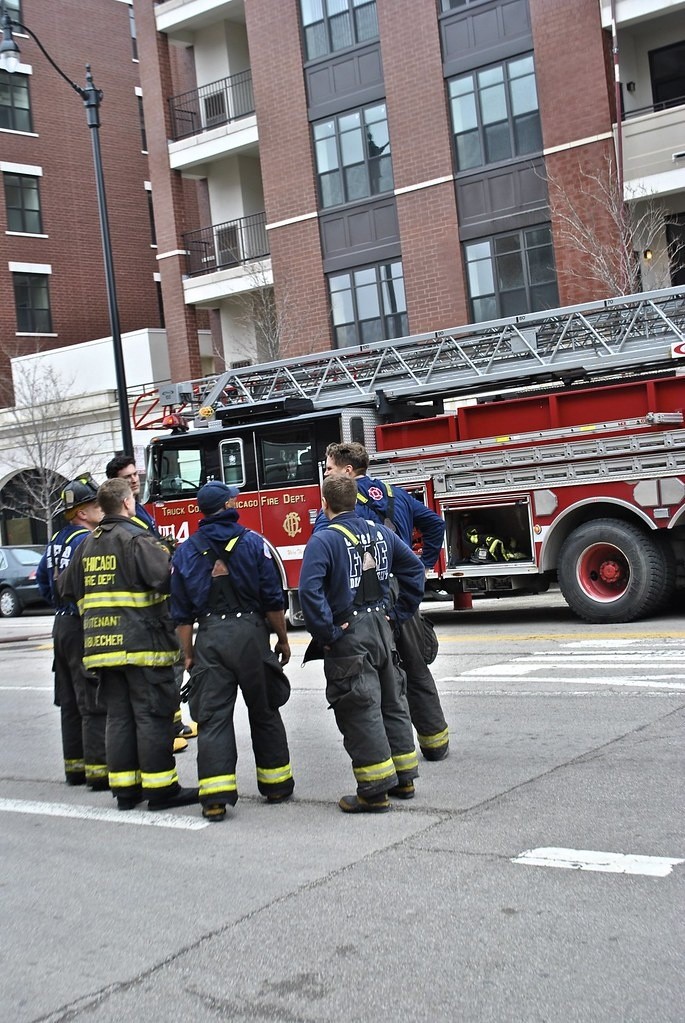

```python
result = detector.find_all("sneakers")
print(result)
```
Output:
[387,776,415,799]
[148,787,199,810]
[116,785,147,810]
[338,790,391,812]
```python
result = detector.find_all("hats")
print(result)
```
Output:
[197,482,240,515]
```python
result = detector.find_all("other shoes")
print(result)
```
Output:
[202,802,227,821]
[65,770,86,786]
[172,738,188,753]
[91,779,110,791]
[267,791,289,803]
[173,721,198,736]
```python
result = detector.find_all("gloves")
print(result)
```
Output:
[178,664,195,703]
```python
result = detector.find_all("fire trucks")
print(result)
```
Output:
[135,284,685,625]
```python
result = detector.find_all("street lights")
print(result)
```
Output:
[0,5,134,458]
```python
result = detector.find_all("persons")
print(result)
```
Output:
[57,478,200,811]
[298,475,426,811]
[106,456,198,753]
[36,483,110,791]
[311,443,449,762]
[171,481,295,821]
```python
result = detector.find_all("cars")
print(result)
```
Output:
[0,545,48,618]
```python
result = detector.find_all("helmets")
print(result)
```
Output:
[470,547,494,564]
[50,472,100,520]
